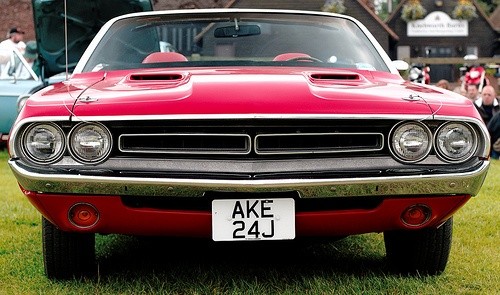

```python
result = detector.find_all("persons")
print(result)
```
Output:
[435,79,500,160]
[0,27,41,81]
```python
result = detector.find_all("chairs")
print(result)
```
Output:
[144,51,189,63]
[274,53,311,62]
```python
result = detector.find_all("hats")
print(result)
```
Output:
[10,28,25,34]
[24,41,39,60]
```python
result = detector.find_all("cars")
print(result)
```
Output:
[7,8,493,284]
